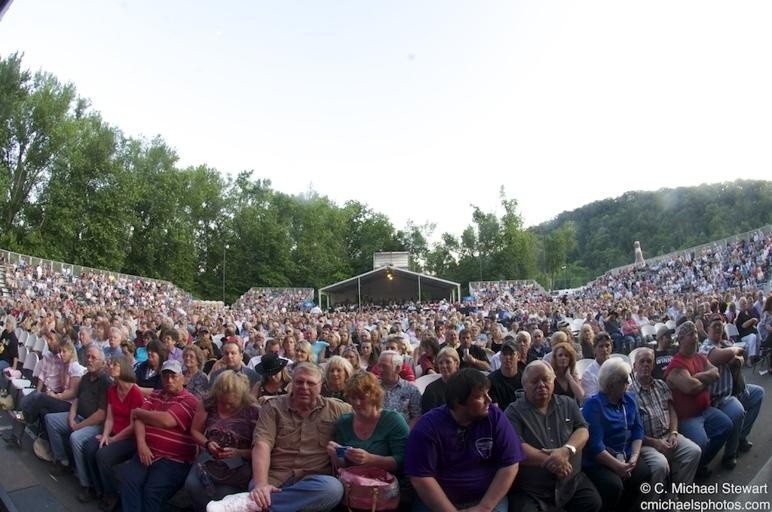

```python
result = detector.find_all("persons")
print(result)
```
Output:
[206,363,353,512]
[0,233,771,470]
[328,370,411,511]
[402,368,526,512]
[185,369,262,510]
[629,347,701,512]
[504,360,602,512]
[120,359,199,511]
[662,321,735,482]
[43,341,112,502]
[581,356,652,511]
[80,354,145,511]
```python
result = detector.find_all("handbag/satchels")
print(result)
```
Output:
[32,432,53,463]
[337,464,400,512]
[197,454,251,500]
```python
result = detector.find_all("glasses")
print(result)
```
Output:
[639,359,653,364]
[293,380,321,387]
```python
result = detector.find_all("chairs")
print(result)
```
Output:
[3,328,50,415]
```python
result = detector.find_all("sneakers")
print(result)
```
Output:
[76,489,95,503]
[723,456,737,468]
[738,440,753,451]
[103,496,118,512]
[206,491,262,512]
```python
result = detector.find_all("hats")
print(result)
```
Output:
[501,341,518,353]
[255,352,288,376]
[199,326,208,333]
[161,360,181,374]
[220,336,238,343]
[656,326,676,340]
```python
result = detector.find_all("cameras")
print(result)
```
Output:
[336,446,351,457]
